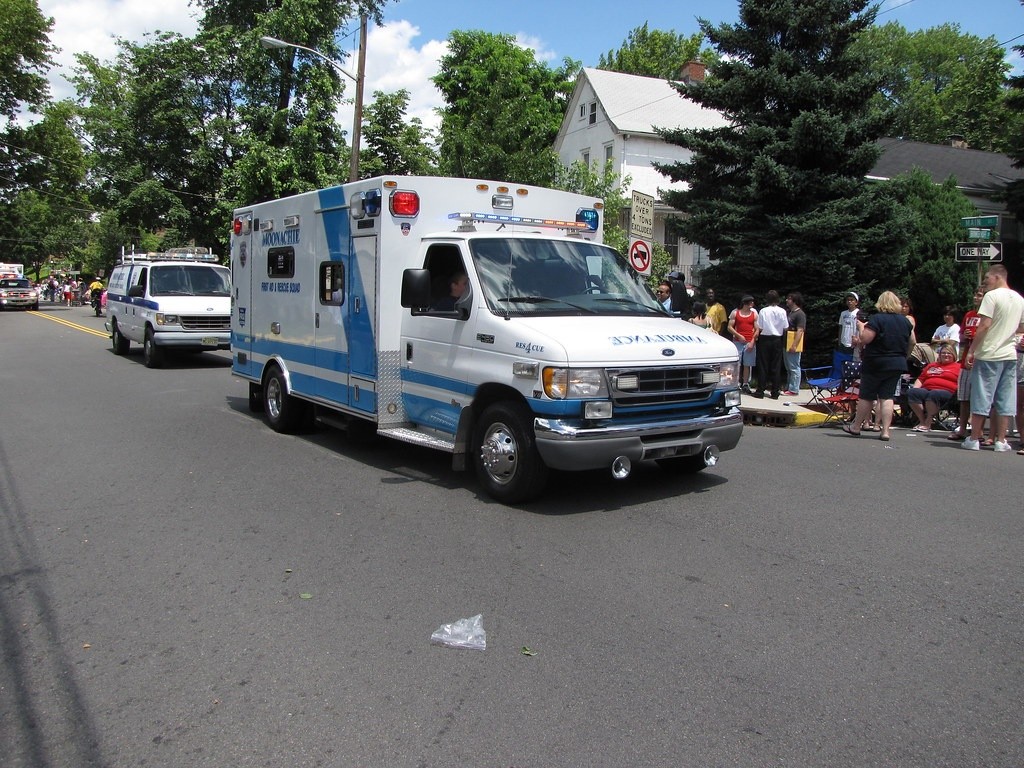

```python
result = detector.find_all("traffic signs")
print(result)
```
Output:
[961,214,1001,240]
[954,241,1002,264]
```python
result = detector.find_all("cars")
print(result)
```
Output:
[0,278,39,311]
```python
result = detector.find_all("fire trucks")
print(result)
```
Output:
[0,263,25,286]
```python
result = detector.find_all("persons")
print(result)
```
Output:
[948,264,1024,455]
[656,271,687,316]
[32,271,91,304]
[842,290,916,441]
[838,292,864,359]
[89,276,105,314]
[931,313,962,361]
[687,288,727,336]
[780,292,806,396]
[728,292,759,393]
[443,272,467,312]
[906,344,961,432]
[752,290,789,399]
[959,285,1024,454]
[898,297,915,331]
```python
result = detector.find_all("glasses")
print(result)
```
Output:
[657,290,666,294]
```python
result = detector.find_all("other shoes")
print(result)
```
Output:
[880,433,890,441]
[751,390,779,400]
[948,433,964,440]
[978,437,995,446]
[742,384,752,395]
[910,424,932,432]
[780,390,798,396]
[861,424,882,432]
[842,425,860,436]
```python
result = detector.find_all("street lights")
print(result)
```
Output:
[260,35,363,184]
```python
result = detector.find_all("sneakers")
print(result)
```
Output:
[961,436,980,451]
[994,439,1012,452]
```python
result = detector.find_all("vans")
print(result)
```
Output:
[105,253,232,368]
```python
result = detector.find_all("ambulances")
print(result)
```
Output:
[228,176,744,500]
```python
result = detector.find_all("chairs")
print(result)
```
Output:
[801,351,971,431]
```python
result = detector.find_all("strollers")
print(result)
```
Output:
[71,290,82,307]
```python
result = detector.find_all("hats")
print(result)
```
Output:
[741,294,754,302]
[665,271,685,282]
[845,292,859,301]
